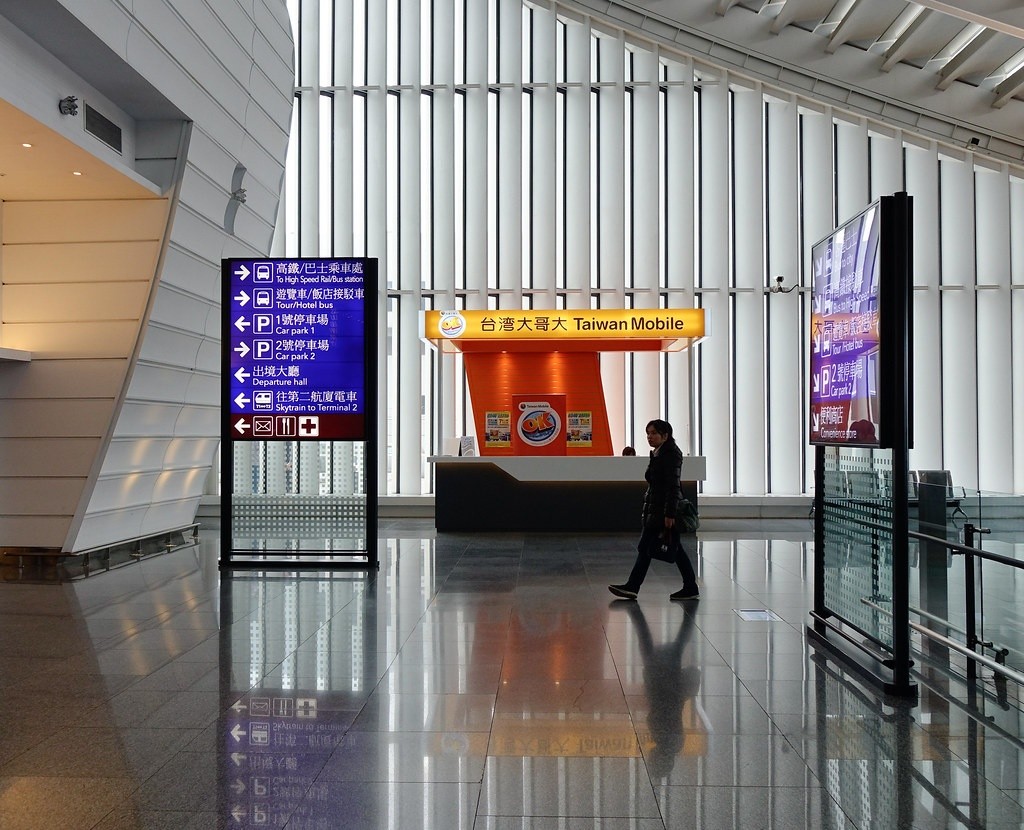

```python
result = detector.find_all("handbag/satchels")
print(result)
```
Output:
[677,499,700,533]
[650,527,678,563]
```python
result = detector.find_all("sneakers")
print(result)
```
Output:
[608,584,640,601]
[670,584,700,600]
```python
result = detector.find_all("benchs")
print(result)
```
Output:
[809,470,969,519]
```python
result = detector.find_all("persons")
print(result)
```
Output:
[608,419,700,601]
[622,447,636,456]
